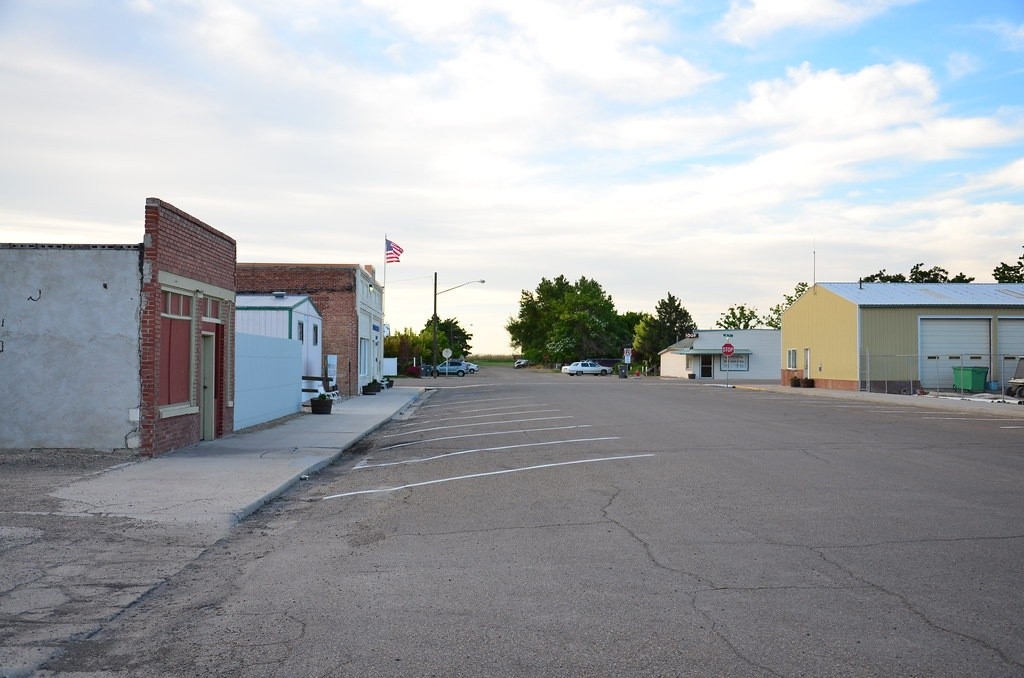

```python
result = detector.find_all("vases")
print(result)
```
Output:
[688,374,696,379]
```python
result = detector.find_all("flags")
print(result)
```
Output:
[386,239,403,264]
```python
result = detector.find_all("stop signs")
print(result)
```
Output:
[722,343,734,356]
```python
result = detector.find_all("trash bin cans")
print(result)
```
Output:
[952,365,989,393]
[619,366,628,379]
[423,365,431,376]
[419,366,425,376]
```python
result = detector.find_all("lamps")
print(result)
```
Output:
[369,283,374,292]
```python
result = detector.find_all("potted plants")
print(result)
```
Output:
[380,377,394,389]
[362,382,377,395]
[310,392,334,415]
[368,379,383,392]
[789,376,801,387]
[800,377,815,388]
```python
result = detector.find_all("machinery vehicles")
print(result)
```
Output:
[1004,358,1024,398]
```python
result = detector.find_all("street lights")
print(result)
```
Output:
[433,270,486,379]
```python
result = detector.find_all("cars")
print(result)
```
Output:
[422,365,434,372]
[514,360,529,369]
[465,362,480,375]
[561,361,613,377]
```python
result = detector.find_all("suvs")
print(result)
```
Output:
[430,359,469,377]
